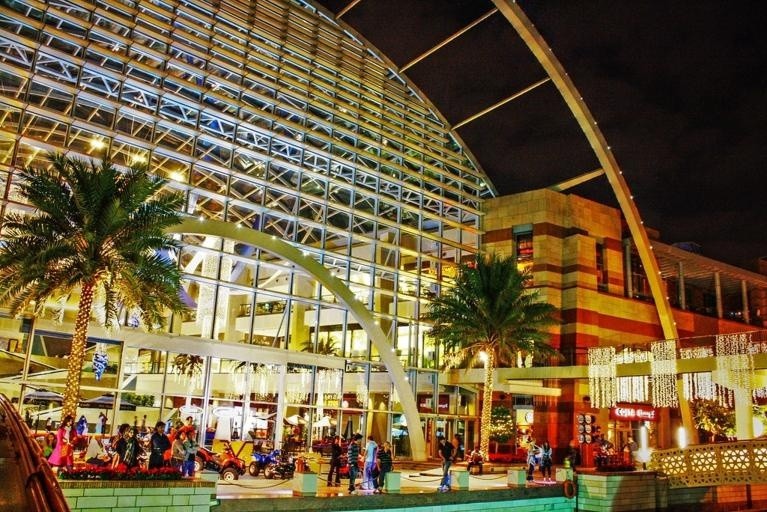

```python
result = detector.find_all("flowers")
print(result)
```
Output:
[62,465,183,481]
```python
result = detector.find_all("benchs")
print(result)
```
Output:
[457,460,530,474]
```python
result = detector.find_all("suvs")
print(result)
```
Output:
[193,439,246,481]
[249,449,296,479]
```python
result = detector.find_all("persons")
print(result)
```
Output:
[467,446,485,475]
[623,434,638,452]
[42,412,196,477]
[538,439,553,482]
[526,450,537,482]
[435,435,455,492]
[326,433,392,494]
